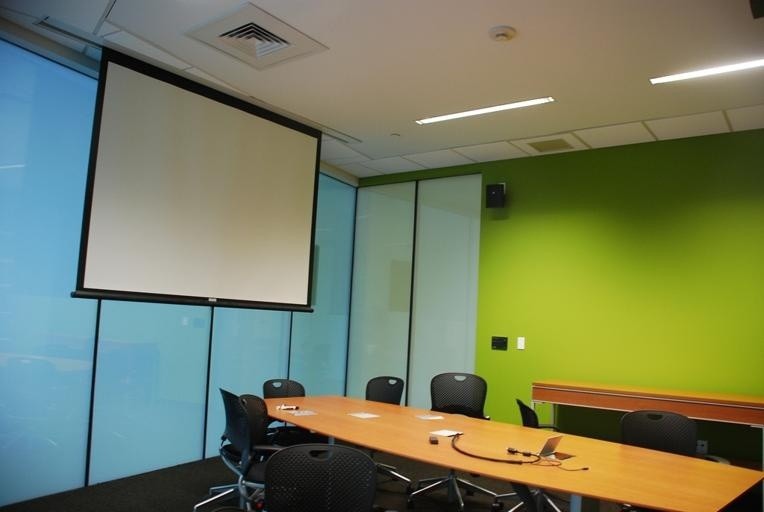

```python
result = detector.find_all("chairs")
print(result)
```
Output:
[357,375,405,473]
[234,391,295,511]
[261,377,311,444]
[193,386,259,511]
[515,397,562,433]
[406,374,499,508]
[612,409,700,462]
[245,441,398,512]
[492,482,569,512]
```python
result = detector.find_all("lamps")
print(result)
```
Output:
[647,59,764,88]
[415,95,558,132]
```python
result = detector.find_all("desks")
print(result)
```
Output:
[248,394,763,512]
[526,375,762,434]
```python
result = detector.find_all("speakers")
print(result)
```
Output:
[486,183,507,209]
